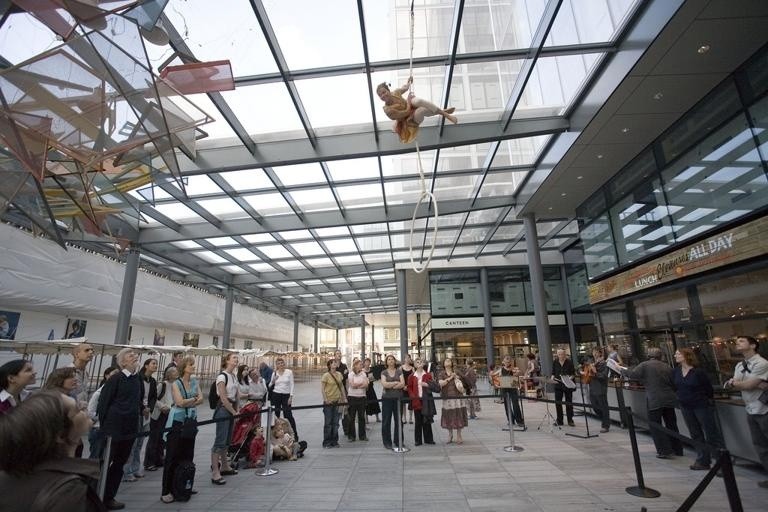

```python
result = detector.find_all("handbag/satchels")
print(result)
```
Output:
[182,422,197,438]
[209,373,227,409]
[455,378,466,394]
[170,460,195,501]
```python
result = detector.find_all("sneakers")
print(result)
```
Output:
[124,465,143,481]
[107,499,125,510]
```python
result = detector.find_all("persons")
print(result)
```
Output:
[727,336,768,489]
[377,77,457,145]
[489,352,540,427]
[322,349,480,450]
[550,349,577,427]
[607,344,623,366]
[620,347,683,458]
[586,346,611,434]
[0,342,307,512]
[670,346,725,479]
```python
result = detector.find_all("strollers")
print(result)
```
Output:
[209,399,265,471]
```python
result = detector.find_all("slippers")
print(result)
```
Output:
[211,477,225,485]
[220,469,237,476]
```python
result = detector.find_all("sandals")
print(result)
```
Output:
[144,465,157,471]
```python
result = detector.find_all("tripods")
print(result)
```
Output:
[537,376,562,433]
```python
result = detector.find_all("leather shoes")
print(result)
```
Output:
[656,448,768,488]
[324,435,463,449]
